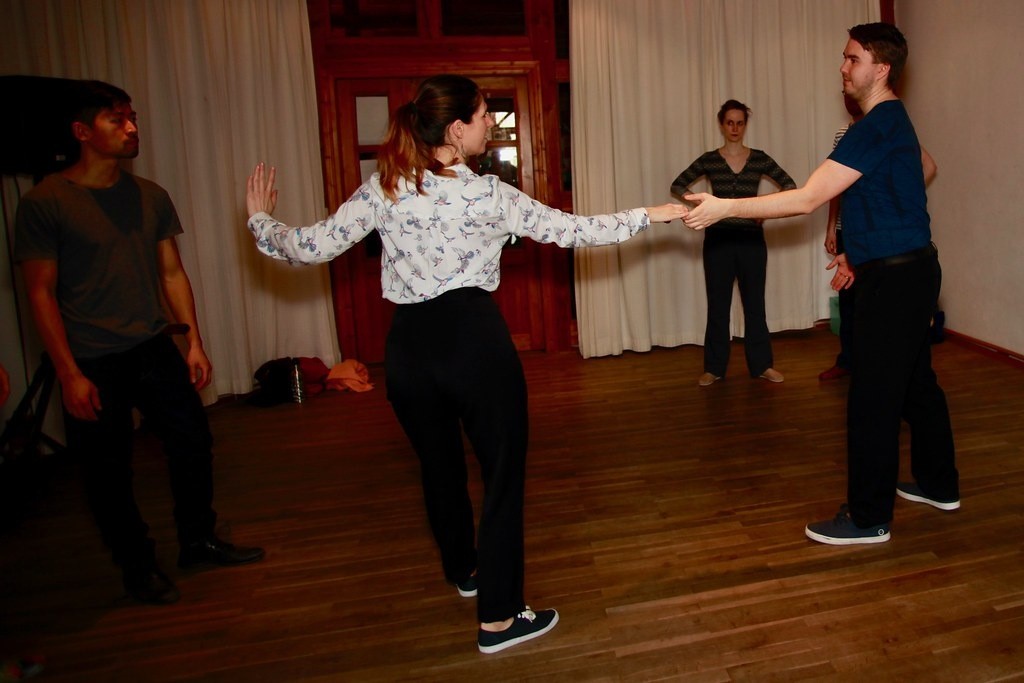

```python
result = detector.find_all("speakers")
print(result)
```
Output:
[0,73,82,180]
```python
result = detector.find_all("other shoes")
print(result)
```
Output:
[761,367,785,383]
[699,372,721,386]
[818,364,847,380]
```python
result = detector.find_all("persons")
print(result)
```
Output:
[248,77,687,647]
[818,88,937,378]
[670,100,796,386]
[15,84,265,605]
[681,22,960,544]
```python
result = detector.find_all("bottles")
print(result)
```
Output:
[292,361,305,403]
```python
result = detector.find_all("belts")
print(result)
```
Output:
[853,243,938,277]
[160,323,192,338]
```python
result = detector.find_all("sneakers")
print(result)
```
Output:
[457,566,478,597]
[896,482,962,511]
[478,606,560,654]
[805,512,891,546]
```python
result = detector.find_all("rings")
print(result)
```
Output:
[845,277,849,279]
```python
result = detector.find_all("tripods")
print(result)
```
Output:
[0,347,72,522]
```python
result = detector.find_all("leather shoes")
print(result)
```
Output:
[133,563,173,601]
[177,523,266,575]
